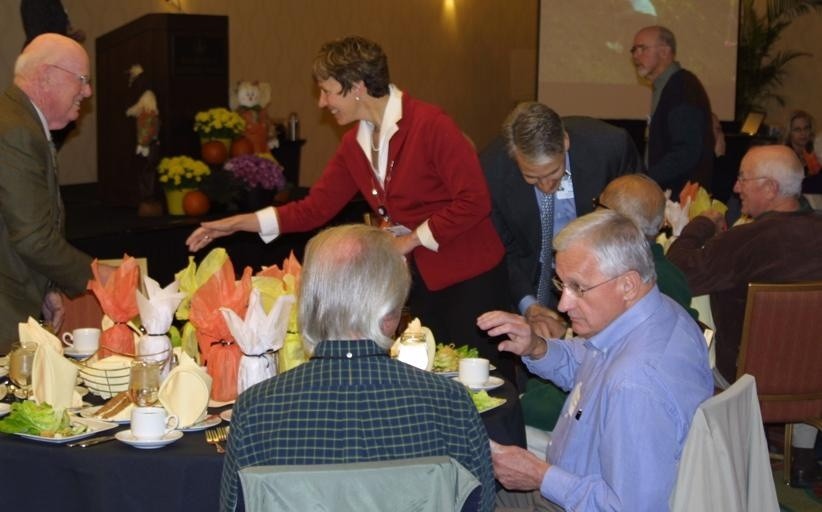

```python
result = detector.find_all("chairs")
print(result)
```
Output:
[715,281,822,485]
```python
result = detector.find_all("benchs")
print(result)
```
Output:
[673,373,780,512]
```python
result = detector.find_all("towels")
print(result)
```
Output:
[32,342,80,413]
[18,316,64,358]
[666,196,691,236]
[155,351,213,429]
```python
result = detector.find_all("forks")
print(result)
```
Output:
[215,425,228,443]
[204,428,226,454]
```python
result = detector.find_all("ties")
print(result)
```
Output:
[536,193,554,306]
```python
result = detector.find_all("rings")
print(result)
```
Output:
[204,235,209,239]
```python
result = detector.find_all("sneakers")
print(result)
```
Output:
[791,463,822,488]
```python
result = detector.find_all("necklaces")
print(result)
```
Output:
[372,142,379,152]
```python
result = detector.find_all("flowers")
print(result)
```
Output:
[157,156,213,188]
[224,153,286,192]
[194,107,245,135]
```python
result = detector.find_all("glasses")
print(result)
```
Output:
[552,270,639,297]
[46,66,90,87]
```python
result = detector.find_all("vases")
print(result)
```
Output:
[201,141,229,164]
[165,188,197,216]
[239,185,278,209]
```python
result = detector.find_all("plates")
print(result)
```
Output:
[431,358,499,377]
[475,397,509,417]
[79,404,142,424]
[112,429,185,450]
[450,376,506,391]
[220,408,233,421]
[13,415,118,444]
[168,410,222,432]
[62,347,98,360]
[0,403,12,417]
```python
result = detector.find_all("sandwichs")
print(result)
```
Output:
[93,392,134,422]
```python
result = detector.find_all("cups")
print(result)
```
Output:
[457,356,489,386]
[127,357,160,407]
[61,328,101,353]
[128,406,178,442]
[396,331,429,370]
[395,307,411,337]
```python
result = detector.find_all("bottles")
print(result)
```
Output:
[168,354,179,372]
[288,112,301,142]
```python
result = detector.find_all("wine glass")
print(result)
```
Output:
[6,339,38,402]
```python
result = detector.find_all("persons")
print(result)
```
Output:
[0,32,116,359]
[629,24,716,202]
[518,174,699,462]
[481,99,647,338]
[782,108,822,196]
[184,34,518,387]
[664,143,822,487]
[478,208,714,512]
[219,221,498,511]
